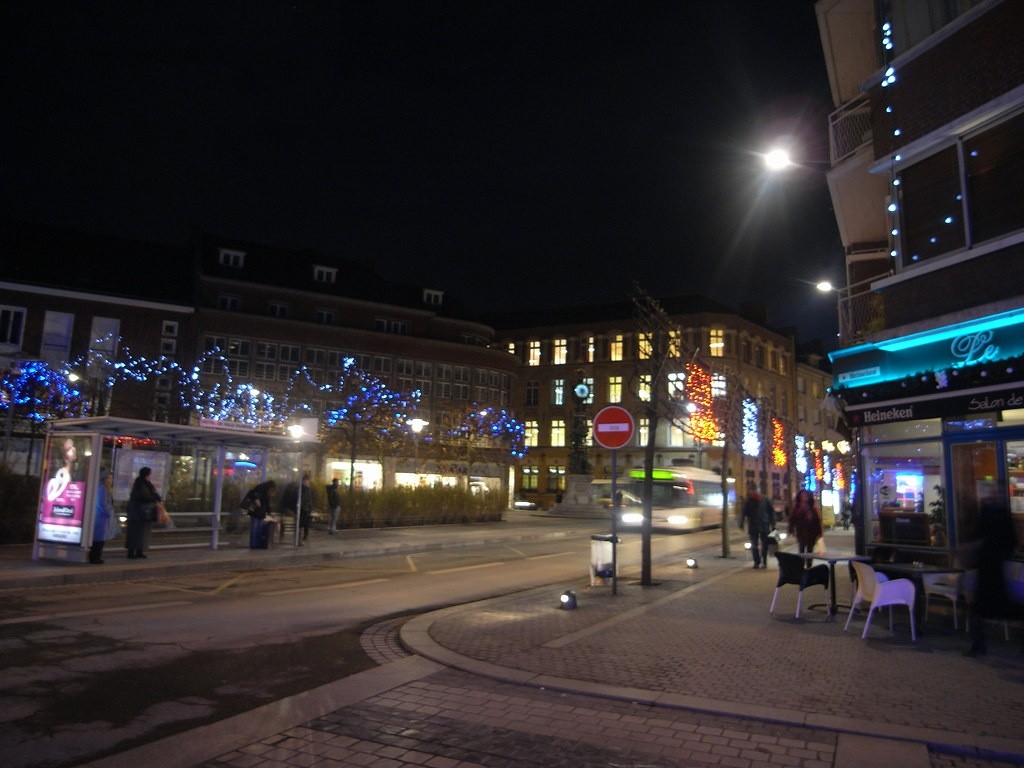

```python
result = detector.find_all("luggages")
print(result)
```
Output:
[260,514,274,549]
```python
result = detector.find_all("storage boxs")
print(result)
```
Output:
[878,510,933,546]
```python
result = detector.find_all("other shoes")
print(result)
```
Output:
[753,560,761,568]
[762,557,766,568]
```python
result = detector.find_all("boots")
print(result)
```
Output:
[126,548,136,559]
[88,542,106,563]
[137,549,147,558]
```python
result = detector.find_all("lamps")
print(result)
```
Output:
[686,556,698,568]
[743,541,753,550]
[560,589,579,610]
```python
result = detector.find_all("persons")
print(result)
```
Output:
[960,503,1018,658]
[123,466,161,558]
[88,466,115,564]
[739,483,776,569]
[292,475,313,539]
[556,491,563,503]
[246,479,276,549]
[616,490,623,507]
[841,502,851,530]
[49,438,77,483]
[326,478,340,533]
[788,489,823,569]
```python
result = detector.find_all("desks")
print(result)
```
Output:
[799,548,876,617]
[871,559,966,635]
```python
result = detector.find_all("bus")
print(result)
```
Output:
[618,466,737,533]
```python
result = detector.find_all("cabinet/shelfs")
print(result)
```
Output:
[1007,470,1024,493]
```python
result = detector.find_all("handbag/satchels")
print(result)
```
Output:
[141,503,155,521]
[812,536,827,556]
[241,486,265,510]
[150,500,170,528]
[767,529,780,556]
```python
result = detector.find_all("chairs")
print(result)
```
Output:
[849,544,900,612]
[767,550,831,624]
[839,559,919,645]
[982,561,1024,640]
[921,572,974,634]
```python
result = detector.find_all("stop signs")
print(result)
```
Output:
[593,406,635,451]
